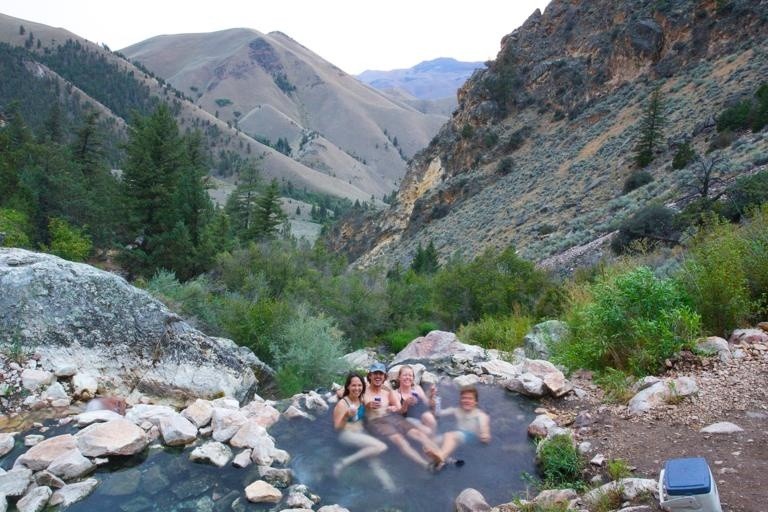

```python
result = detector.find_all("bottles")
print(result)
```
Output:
[433,393,442,418]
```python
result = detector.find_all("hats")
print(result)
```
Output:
[367,363,388,375]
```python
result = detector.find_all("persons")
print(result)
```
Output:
[419,370,445,413]
[390,363,440,438]
[329,372,399,498]
[418,383,494,465]
[334,360,465,477]
